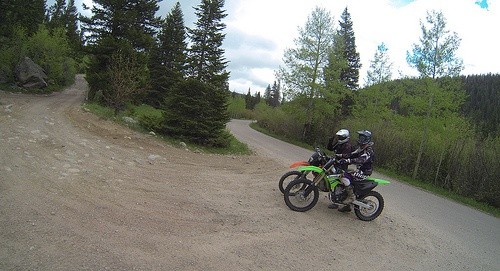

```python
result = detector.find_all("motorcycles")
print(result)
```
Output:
[278,145,334,195]
[285,150,391,220]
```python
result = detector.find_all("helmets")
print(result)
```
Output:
[335,129,350,144]
[356,130,372,145]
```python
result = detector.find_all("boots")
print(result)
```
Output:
[338,205,352,212]
[343,184,356,204]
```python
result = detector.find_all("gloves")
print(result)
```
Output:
[327,136,335,150]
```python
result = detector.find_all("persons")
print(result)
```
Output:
[327,128,352,210]
[333,129,376,214]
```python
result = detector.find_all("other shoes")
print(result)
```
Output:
[329,204,338,209]
